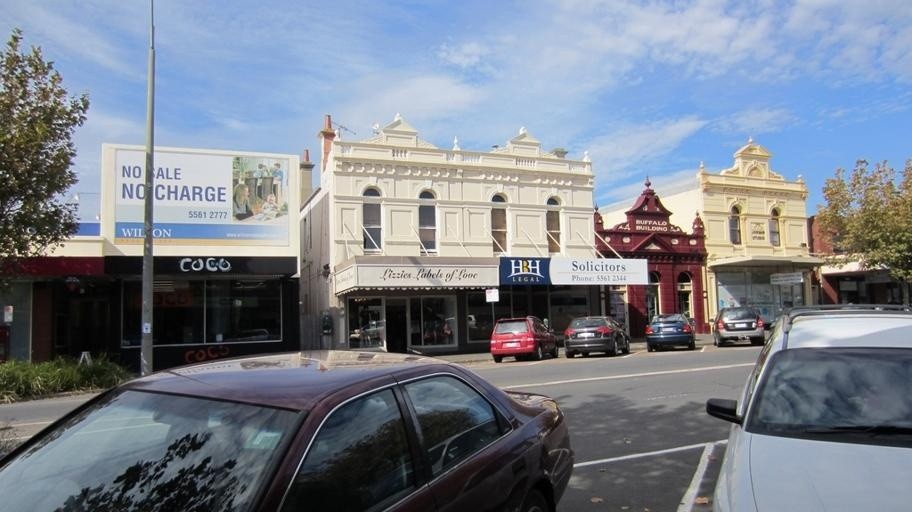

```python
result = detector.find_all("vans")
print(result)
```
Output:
[468,314,480,336]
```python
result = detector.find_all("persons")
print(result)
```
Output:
[270,162,284,205]
[232,183,254,223]
[252,163,266,187]
[319,308,333,350]
[260,193,280,213]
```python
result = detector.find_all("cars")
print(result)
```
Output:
[489,306,765,364]
[349,319,385,340]
[0,349,575,511]
[704,304,912,510]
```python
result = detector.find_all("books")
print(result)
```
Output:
[251,208,280,224]
